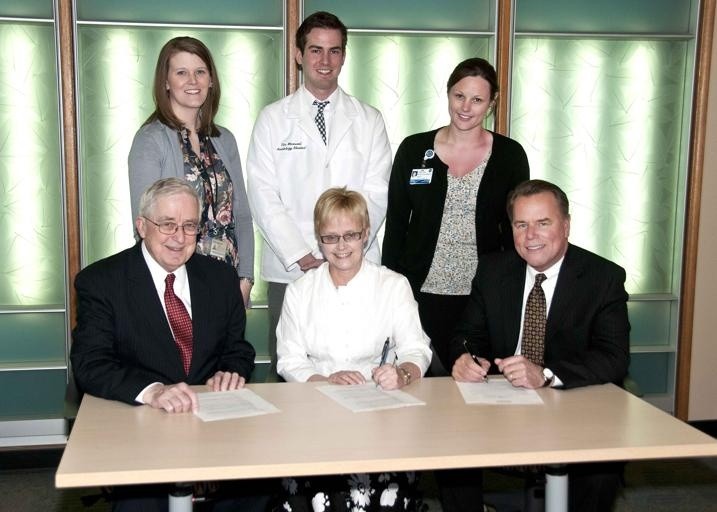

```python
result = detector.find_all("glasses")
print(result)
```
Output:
[142,216,200,235]
[318,228,363,243]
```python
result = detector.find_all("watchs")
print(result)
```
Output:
[541,367,555,390]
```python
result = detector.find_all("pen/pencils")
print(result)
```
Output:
[375,337,391,387]
[464,340,489,383]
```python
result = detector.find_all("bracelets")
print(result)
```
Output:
[401,369,411,385]
[241,277,255,287]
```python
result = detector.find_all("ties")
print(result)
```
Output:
[521,273,549,369]
[164,272,194,378]
[312,99,329,145]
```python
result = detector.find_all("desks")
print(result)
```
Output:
[54,374,717,512]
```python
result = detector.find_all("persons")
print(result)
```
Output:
[382,55,531,512]
[434,177,632,512]
[67,177,258,511]
[126,34,256,309]
[274,185,434,512]
[243,8,394,383]
